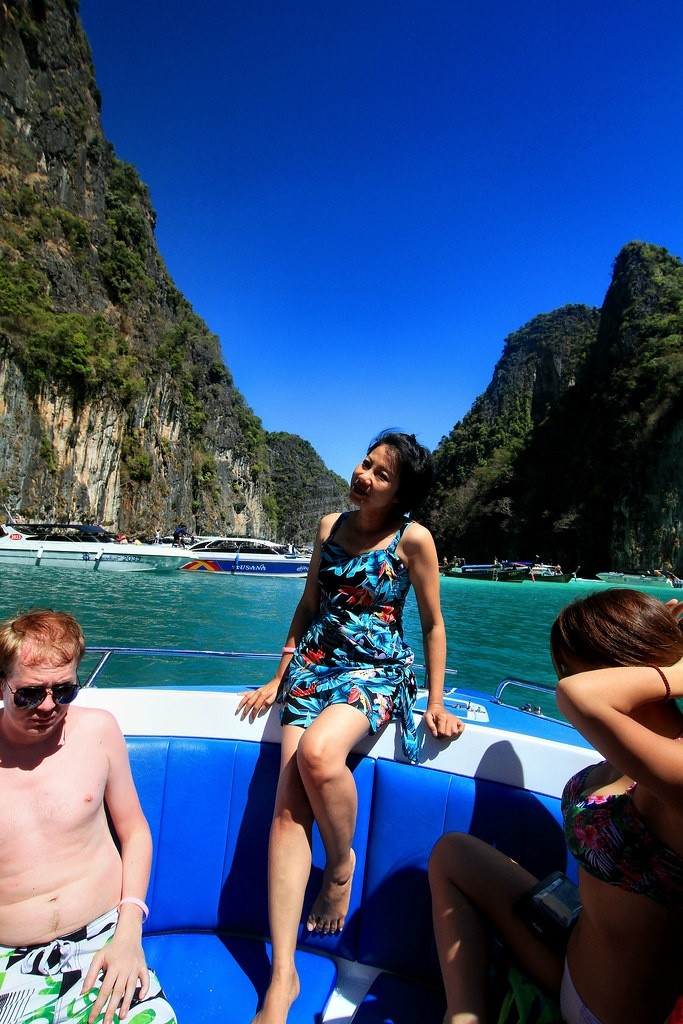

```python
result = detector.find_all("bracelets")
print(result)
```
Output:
[117,896,149,923]
[643,662,671,700]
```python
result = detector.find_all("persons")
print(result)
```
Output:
[0,610,178,1024]
[654,570,662,576]
[442,556,457,567]
[429,587,683,1024]
[116,531,143,545]
[234,431,465,1024]
[154,526,162,543]
[172,524,193,549]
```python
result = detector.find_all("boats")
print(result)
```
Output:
[179,537,312,578]
[595,570,683,589]
[439,556,539,583]
[0,523,199,574]
[525,565,581,583]
[148,534,312,559]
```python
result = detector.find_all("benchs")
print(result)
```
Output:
[130,736,578,1024]
